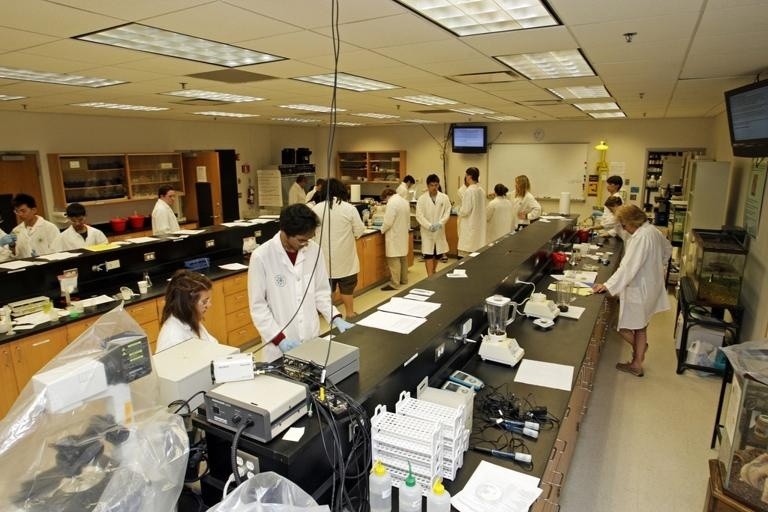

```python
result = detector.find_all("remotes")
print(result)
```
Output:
[440,380,478,397]
[448,370,486,393]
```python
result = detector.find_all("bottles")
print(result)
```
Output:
[368,457,451,512]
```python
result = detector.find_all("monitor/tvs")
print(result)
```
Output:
[452,126,487,153]
[724,78,768,158]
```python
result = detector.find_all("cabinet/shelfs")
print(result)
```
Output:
[0,325,68,422]
[368,152,406,185]
[46,152,128,207]
[530,297,615,512]
[223,273,260,346]
[377,232,414,282]
[123,295,159,356]
[130,152,186,201]
[336,151,368,184]
[157,277,227,346]
[353,233,375,293]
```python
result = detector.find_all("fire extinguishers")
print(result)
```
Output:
[248,185,255,204]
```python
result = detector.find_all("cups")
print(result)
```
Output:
[137,280,148,294]
[557,280,579,312]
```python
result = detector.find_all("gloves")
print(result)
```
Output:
[334,318,353,333]
[0,233,16,246]
[430,223,440,231]
[279,340,300,352]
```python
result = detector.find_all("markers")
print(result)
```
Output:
[473,446,532,464]
[489,417,539,430]
[499,423,538,439]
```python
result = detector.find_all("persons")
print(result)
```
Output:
[247,203,356,364]
[10,192,60,259]
[51,202,108,255]
[380,189,411,291]
[457,167,488,256]
[488,175,541,229]
[309,179,326,203]
[592,204,674,376]
[583,176,632,243]
[312,178,365,323]
[0,227,20,263]
[416,185,448,263]
[415,174,452,277]
[155,269,219,352]
[487,183,513,242]
[288,175,318,205]
[396,175,415,200]
[152,185,181,235]
[456,176,470,237]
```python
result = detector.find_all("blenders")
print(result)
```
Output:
[478,294,525,367]
[523,292,560,321]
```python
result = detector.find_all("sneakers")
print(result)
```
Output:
[633,342,648,361]
[381,285,398,290]
[616,362,644,376]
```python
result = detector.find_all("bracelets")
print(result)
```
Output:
[525,213,527,219]
